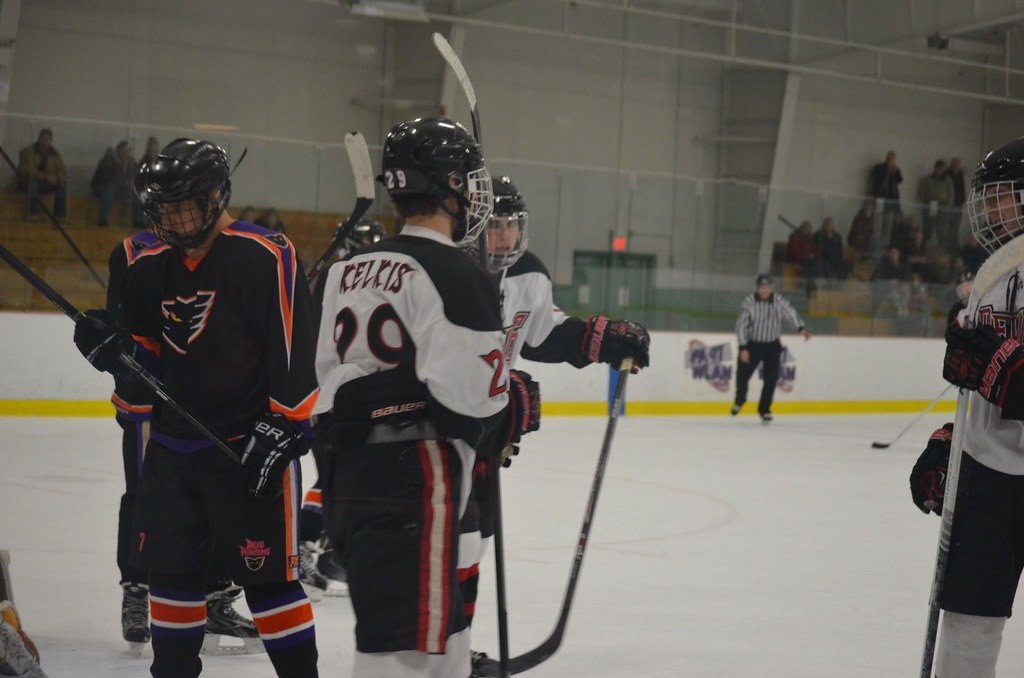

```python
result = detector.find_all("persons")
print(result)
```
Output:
[12,128,67,217]
[238,206,255,223]
[730,272,809,425]
[138,135,160,165]
[70,113,652,678]
[908,138,1024,678]
[787,151,991,341]
[92,139,146,229]
[255,208,285,233]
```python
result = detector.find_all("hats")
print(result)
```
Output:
[757,274,773,288]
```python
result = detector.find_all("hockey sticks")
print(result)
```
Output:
[302,130,378,306]
[0,147,252,470]
[869,233,1024,678]
[468,354,634,678]
[428,32,512,678]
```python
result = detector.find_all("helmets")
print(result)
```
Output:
[470,173,529,273]
[970,139,1024,257]
[332,215,385,259]
[383,116,493,247]
[134,138,231,248]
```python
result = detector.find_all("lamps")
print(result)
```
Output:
[941,37,1002,54]
[344,0,430,23]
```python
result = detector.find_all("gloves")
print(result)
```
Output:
[75,308,138,380]
[943,322,1024,407]
[910,423,955,517]
[245,413,309,503]
[581,314,650,374]
[500,367,540,443]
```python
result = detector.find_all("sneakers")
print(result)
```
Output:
[315,546,351,597]
[293,540,327,601]
[201,584,268,655]
[469,648,490,677]
[760,407,772,424]
[120,577,151,659]
[730,403,742,415]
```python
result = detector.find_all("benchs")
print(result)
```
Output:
[772,247,966,337]
[0,191,396,310]
